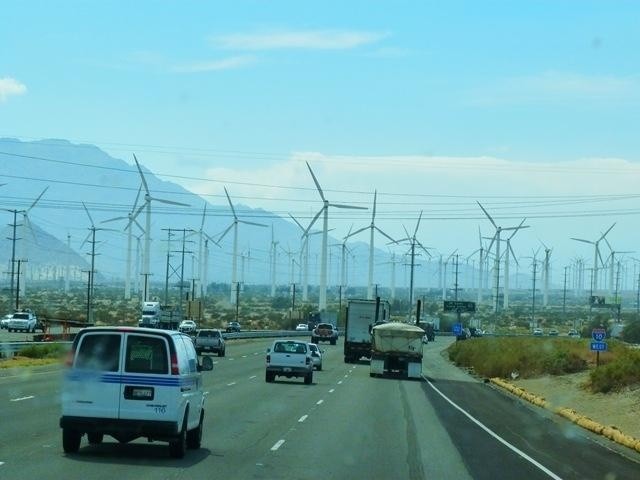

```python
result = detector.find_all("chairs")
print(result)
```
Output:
[277,344,305,353]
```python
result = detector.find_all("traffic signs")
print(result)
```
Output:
[591,329,606,342]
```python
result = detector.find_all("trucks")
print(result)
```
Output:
[344,297,436,380]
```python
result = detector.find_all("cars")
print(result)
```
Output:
[60,302,241,458]
[457,328,483,340]
[265,323,338,383]
[534,328,577,335]
[1,310,37,333]
